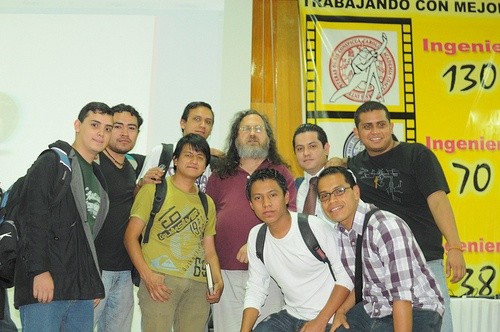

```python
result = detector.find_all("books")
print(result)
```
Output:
[205,264,214,295]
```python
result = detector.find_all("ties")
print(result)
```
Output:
[302,177,318,215]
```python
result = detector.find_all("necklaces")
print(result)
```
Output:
[104,148,124,165]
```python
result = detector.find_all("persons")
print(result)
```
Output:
[205,108,298,332]
[240,168,355,332]
[324,100,466,332]
[133,101,227,200]
[93,104,146,332]
[123,133,224,332]
[315,166,446,332]
[13,101,114,332]
[292,123,357,220]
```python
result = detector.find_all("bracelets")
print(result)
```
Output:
[138,178,143,188]
[448,246,464,253]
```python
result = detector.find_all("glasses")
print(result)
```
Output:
[239,125,264,134]
[318,187,354,202]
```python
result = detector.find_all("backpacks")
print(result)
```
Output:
[131,178,208,287]
[0,149,64,288]
